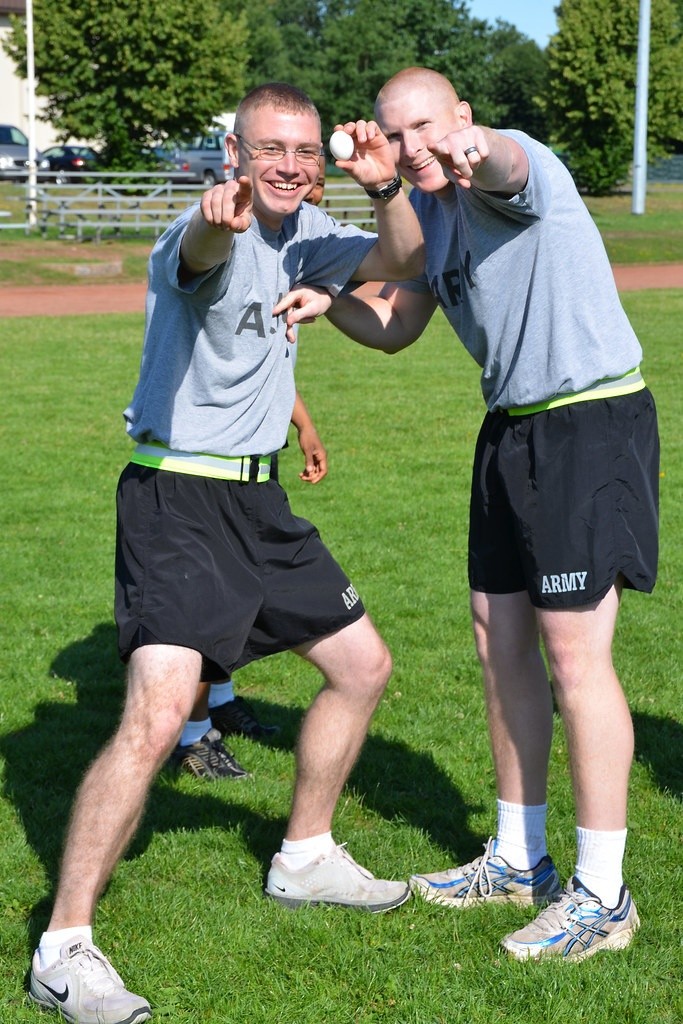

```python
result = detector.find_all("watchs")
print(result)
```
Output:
[364,171,402,200]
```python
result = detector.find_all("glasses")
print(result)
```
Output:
[233,132,323,164]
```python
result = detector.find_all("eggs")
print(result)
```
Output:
[329,130,355,161]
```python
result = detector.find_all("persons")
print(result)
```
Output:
[169,149,326,782]
[271,68,661,965]
[28,82,426,1024]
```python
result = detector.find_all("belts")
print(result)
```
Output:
[509,367,646,417]
[130,441,272,483]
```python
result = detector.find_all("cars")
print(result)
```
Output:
[0,125,49,181]
[38,147,111,183]
[149,131,239,184]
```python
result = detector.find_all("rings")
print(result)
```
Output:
[464,147,478,156]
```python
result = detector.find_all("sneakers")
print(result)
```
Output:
[29,936,151,1024]
[409,836,562,907]
[169,728,248,781]
[500,875,641,964]
[265,841,410,913]
[207,695,282,747]
